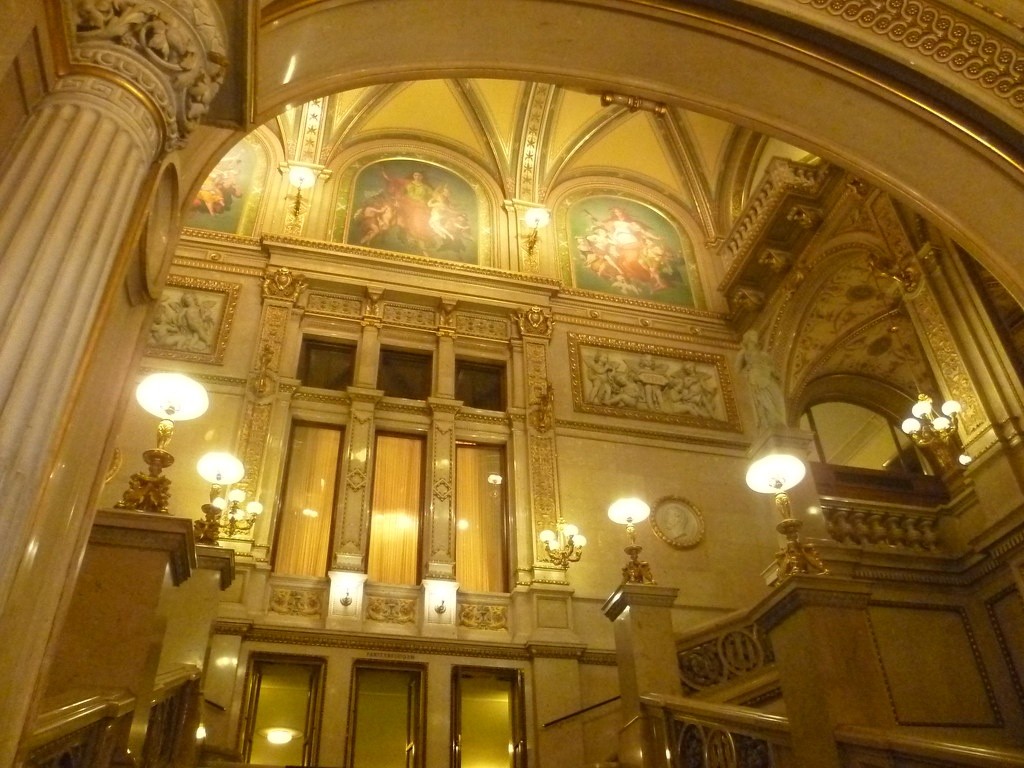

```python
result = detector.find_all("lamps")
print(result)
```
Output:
[745,454,831,589]
[212,344,274,537]
[535,382,586,570]
[524,208,548,255]
[193,451,244,548]
[866,254,960,470]
[114,373,209,517]
[607,497,657,586]
[289,166,314,216]
